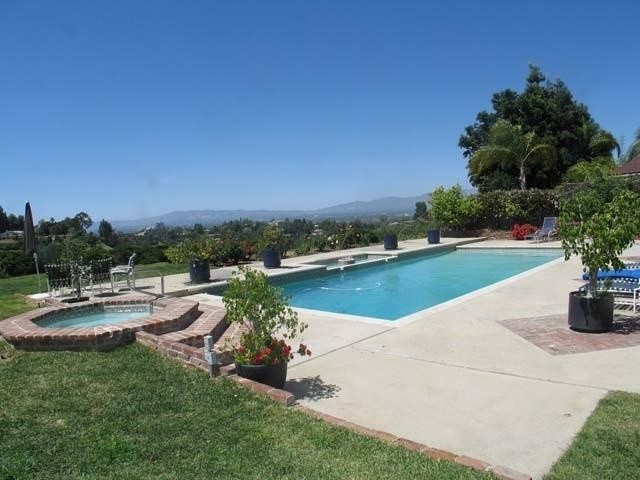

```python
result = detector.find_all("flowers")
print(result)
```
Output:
[216,263,313,365]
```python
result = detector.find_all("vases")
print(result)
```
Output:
[235,366,288,390]
[427,229,441,244]
[383,232,398,249]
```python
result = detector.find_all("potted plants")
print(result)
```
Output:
[553,155,640,333]
[163,236,227,281]
[256,225,292,269]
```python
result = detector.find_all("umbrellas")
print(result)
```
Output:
[22,200,45,293]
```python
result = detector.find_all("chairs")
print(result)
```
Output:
[576,261,640,314]
[523,216,558,243]
[43,252,137,301]
